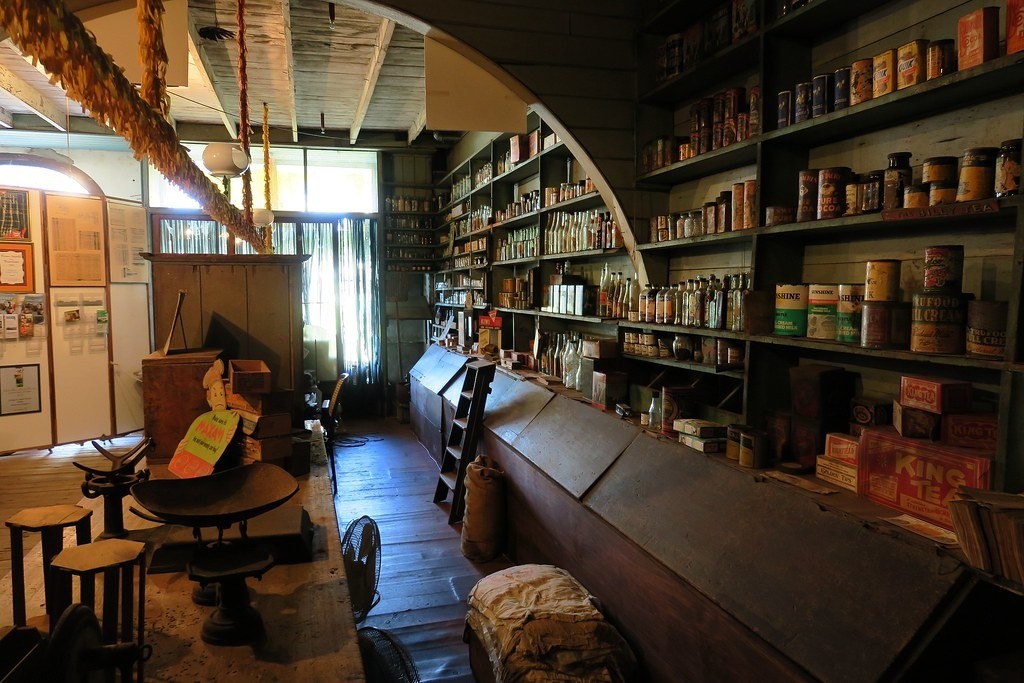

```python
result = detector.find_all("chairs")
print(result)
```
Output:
[321,372,349,494]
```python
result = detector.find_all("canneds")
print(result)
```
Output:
[497,189,539,222]
[623,329,743,364]
[678,84,760,160]
[499,278,528,310]
[657,179,756,242]
[766,138,1024,226]
[774,245,1009,361]
[544,179,598,207]
[776,38,956,129]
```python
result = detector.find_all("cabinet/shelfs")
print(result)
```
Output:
[382,0,1024,493]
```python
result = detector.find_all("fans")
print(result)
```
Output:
[341,514,382,625]
[358,626,421,683]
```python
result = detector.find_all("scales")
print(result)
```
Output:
[129,463,300,644]
[73,436,313,574]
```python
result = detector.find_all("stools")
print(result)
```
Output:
[48,538,150,683]
[4,504,94,626]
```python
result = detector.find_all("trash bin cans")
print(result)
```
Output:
[397,382,411,424]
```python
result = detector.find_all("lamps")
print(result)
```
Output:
[253,208,274,241]
[204,142,248,197]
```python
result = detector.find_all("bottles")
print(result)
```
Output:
[385,192,445,212]
[673,333,692,361]
[471,204,491,231]
[385,214,433,229]
[596,267,749,332]
[544,211,623,256]
[677,211,702,239]
[556,260,571,275]
[387,229,436,258]
[537,329,593,392]
[475,150,512,188]
[648,391,661,431]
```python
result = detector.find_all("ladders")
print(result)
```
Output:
[433,360,496,524]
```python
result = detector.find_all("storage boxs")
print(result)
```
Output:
[478,285,994,532]
[1004,0,1024,56]
[224,359,292,472]
[957,6,1001,72]
[509,135,529,164]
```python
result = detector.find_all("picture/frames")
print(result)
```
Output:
[0,241,36,295]
[0,189,31,242]
[0,363,42,416]
